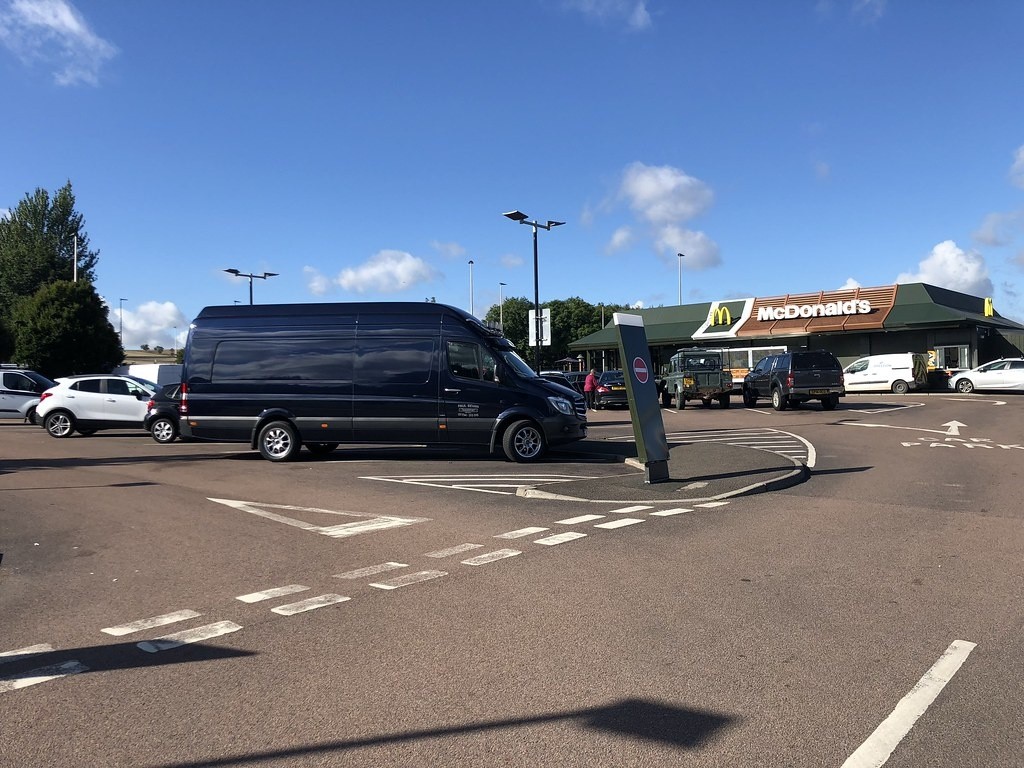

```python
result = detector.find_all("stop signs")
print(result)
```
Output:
[633,357,648,384]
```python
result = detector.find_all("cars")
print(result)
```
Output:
[144,384,188,443]
[565,371,602,401]
[595,371,628,410]
[948,356,1024,393]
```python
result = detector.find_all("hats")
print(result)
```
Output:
[591,369,596,372]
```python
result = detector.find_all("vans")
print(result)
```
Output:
[842,352,927,394]
[181,302,588,462]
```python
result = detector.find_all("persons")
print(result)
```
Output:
[584,369,598,411]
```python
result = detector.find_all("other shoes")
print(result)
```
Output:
[587,408,590,411]
[591,408,595,410]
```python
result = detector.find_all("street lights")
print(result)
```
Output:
[223,269,279,305]
[504,211,566,377]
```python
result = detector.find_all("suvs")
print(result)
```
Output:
[36,374,163,438]
[0,364,58,425]
[743,349,846,410]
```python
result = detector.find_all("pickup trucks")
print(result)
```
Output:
[660,350,733,409]
